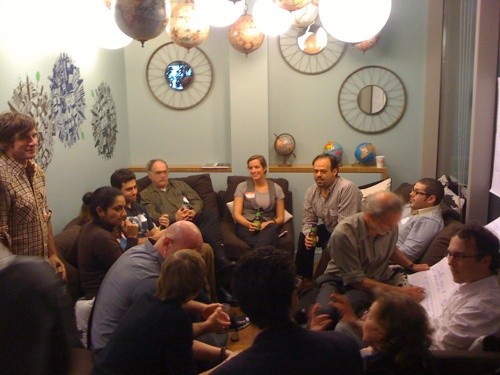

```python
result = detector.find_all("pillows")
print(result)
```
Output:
[358,176,392,201]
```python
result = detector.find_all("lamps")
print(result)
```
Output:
[84,0,393,57]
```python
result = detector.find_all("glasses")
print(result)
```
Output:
[363,311,385,327]
[447,250,476,262]
[411,187,425,195]
[151,170,169,176]
[294,275,303,290]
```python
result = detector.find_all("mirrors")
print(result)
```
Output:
[277,15,348,76]
[337,65,408,136]
[145,41,214,112]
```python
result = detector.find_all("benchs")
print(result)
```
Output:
[393,180,463,275]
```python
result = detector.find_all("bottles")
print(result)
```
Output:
[252,211,261,232]
[309,224,317,249]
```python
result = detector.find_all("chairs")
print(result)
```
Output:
[137,174,224,259]
[53,227,84,270]
[226,177,295,253]
[60,216,89,231]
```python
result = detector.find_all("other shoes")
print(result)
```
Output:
[216,312,250,335]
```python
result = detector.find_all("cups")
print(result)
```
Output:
[376,155,384,169]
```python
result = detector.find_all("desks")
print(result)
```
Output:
[269,163,389,181]
[128,164,232,173]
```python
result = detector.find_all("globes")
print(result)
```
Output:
[272,132,297,168]
[355,144,378,165]
[323,142,342,166]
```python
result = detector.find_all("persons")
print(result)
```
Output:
[309,281,440,375]
[294,154,363,299]
[78,159,215,296]
[208,246,364,375]
[0,113,65,284]
[233,154,285,289]
[0,261,86,375]
[429,220,500,352]
[86,220,231,352]
[105,249,243,375]
[317,178,445,336]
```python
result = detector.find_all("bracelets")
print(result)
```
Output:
[219,346,227,362]
[273,218,277,225]
